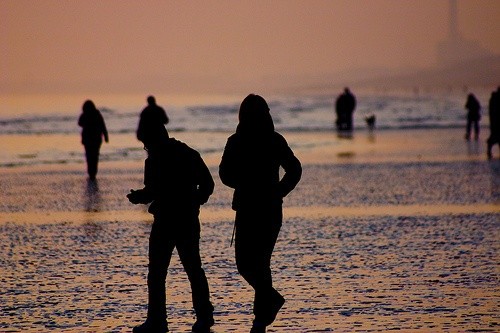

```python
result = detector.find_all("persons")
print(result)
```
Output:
[138,95,169,127]
[77,100,110,180]
[334,87,357,131]
[463,93,482,141]
[486,84,500,158]
[218,92,302,333]
[126,116,217,333]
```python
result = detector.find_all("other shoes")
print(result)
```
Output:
[190,316,214,333]
[261,290,285,326]
[250,318,266,333]
[133,320,169,333]
